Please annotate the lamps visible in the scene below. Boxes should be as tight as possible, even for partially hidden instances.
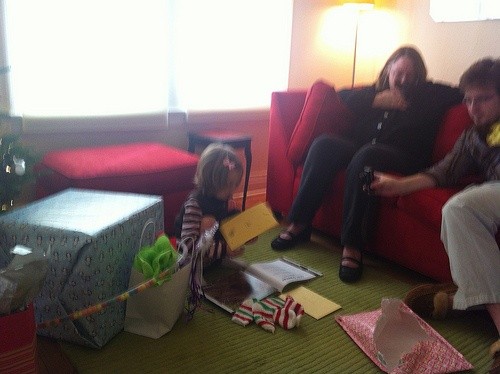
[342,0,376,89]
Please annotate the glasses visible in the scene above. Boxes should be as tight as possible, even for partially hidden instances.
[459,93,496,106]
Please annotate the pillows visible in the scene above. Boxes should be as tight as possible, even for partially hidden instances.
[287,79,356,164]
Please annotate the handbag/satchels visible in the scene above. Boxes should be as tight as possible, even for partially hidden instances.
[0,305,37,374]
[123,219,194,338]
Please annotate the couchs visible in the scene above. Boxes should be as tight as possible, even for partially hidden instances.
[266,79,476,286]
[33,142,199,242]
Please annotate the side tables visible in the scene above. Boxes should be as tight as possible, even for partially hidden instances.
[187,128,253,212]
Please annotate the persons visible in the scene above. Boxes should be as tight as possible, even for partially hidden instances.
[269,47,464,284]
[170,143,259,271]
[371,57,500,374]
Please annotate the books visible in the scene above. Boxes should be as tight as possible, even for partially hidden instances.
[198,254,322,313]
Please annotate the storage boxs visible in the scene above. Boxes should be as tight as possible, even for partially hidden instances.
[0,186,164,351]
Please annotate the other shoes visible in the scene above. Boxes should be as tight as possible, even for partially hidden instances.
[338,253,364,282]
[271,226,310,250]
